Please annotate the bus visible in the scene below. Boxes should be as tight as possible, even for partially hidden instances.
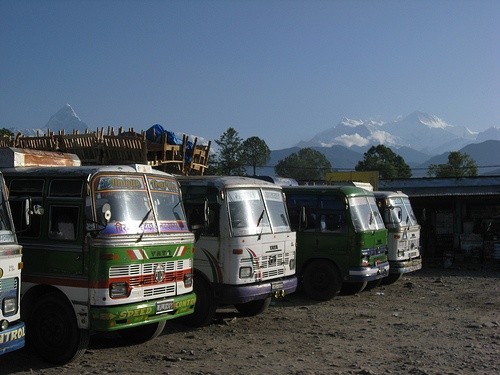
[0,173,26,355]
[179,175,297,317]
[0,146,196,365]
[245,176,390,296]
[373,191,422,283]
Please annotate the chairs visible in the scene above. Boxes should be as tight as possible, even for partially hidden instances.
[0,126,212,177]
